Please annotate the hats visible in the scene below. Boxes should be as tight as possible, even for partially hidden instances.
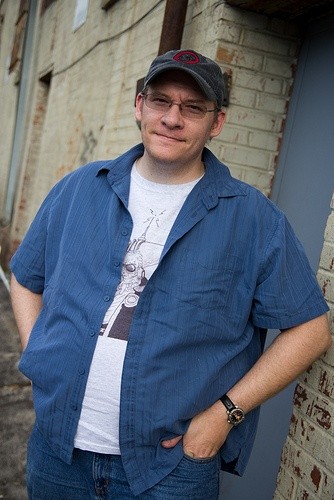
[143,48,226,112]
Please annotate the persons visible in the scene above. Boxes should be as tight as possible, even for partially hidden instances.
[9,50,332,499]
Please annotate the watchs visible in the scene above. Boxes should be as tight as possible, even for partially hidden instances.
[220,395,245,425]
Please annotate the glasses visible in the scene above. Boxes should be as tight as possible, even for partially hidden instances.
[142,91,222,120]
